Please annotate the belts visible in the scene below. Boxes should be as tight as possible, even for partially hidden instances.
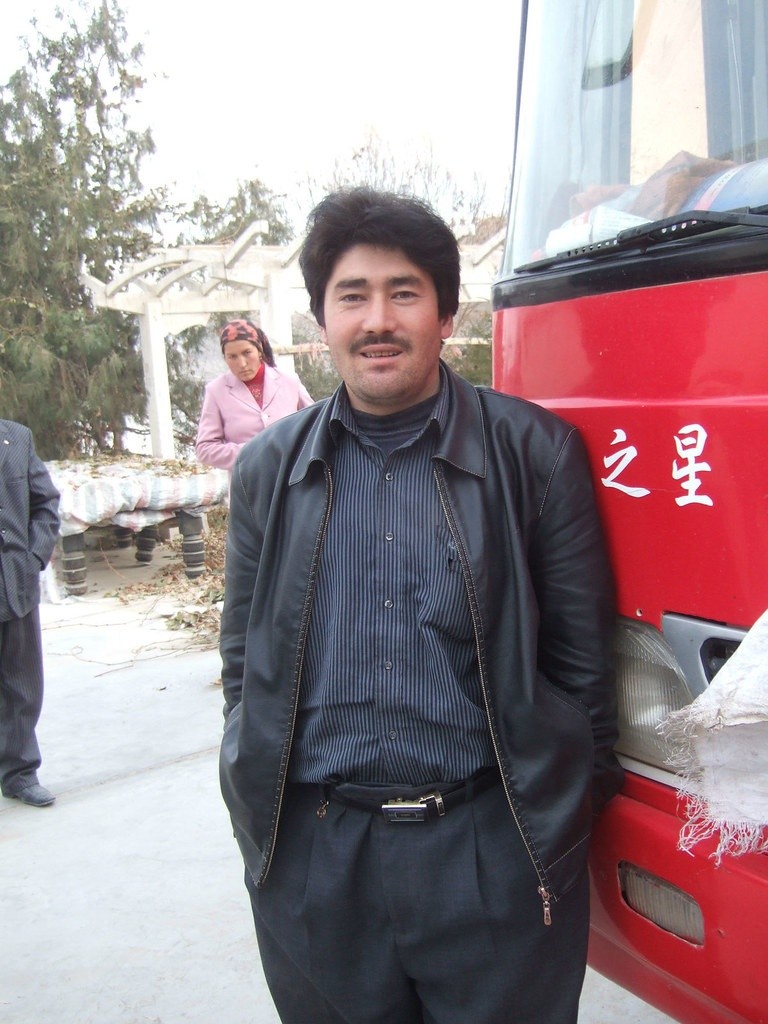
[324,764,502,822]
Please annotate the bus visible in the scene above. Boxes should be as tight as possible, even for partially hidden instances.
[491,2,768,1024]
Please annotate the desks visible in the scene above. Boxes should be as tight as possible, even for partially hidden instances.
[45,449,228,597]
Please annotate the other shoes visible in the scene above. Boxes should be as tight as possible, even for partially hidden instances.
[3,783,56,807]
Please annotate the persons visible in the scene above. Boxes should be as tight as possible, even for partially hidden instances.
[0,418,60,806]
[195,320,314,508]
[195,185,625,1024]
